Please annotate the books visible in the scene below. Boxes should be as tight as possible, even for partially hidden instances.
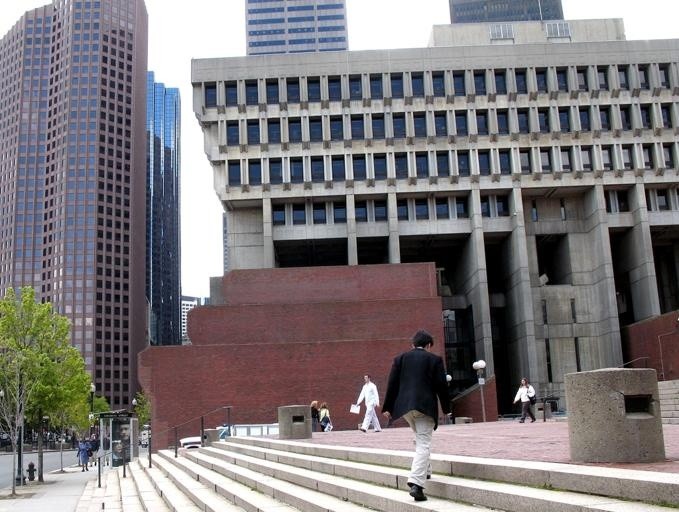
[349,403,361,415]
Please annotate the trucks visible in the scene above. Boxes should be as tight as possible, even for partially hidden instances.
[179,423,279,450]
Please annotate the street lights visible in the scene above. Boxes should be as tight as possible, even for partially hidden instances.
[88,382,96,413]
[0,390,5,402]
[88,413,94,437]
[132,397,138,412]
[472,360,488,422]
[445,373,456,424]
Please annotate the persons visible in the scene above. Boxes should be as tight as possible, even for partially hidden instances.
[513,377,537,423]
[318,402,333,432]
[355,373,383,433]
[120,422,130,441]
[70,431,111,472]
[112,439,124,461]
[381,329,454,502]
[310,400,320,432]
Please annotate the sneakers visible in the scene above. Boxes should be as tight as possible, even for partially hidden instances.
[361,428,380,432]
[410,486,426,501]
[531,418,536,422]
[427,475,430,479]
[82,462,96,471]
[520,419,524,423]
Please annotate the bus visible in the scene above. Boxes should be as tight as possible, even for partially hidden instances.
[139,424,151,447]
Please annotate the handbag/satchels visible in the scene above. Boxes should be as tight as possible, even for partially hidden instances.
[323,416,329,423]
[87,449,93,457]
[530,395,536,404]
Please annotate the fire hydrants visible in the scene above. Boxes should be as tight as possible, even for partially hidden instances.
[25,462,37,481]
